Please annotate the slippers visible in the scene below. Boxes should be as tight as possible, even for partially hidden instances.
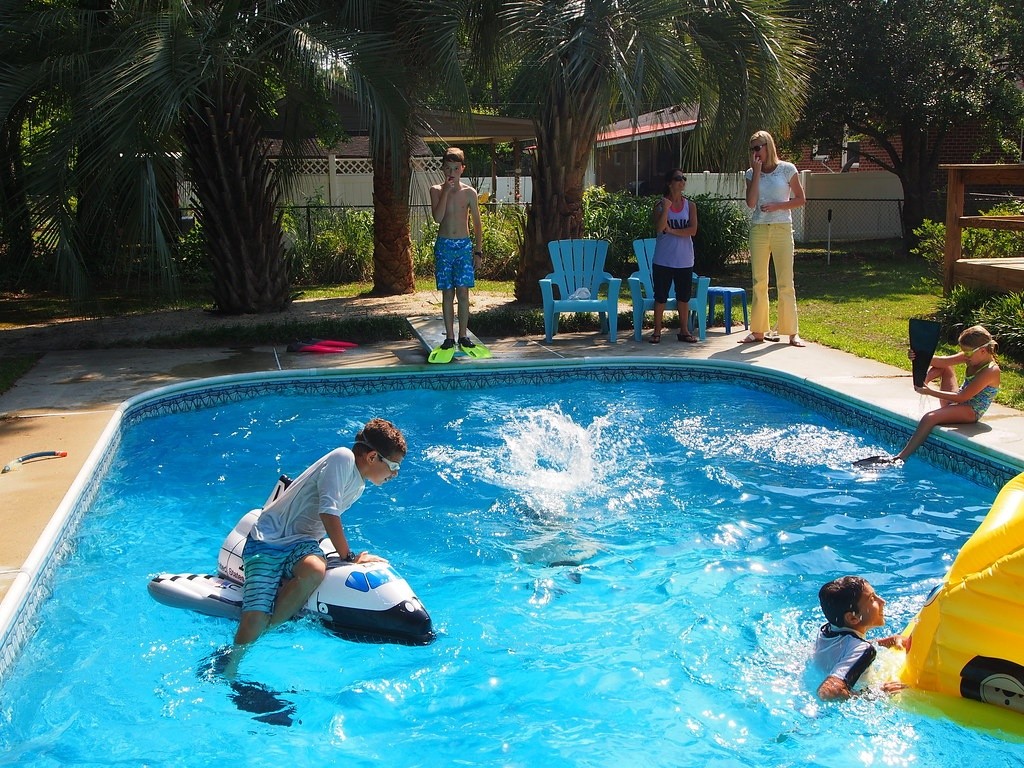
[678,335,697,343]
[649,334,660,343]
[763,330,780,341]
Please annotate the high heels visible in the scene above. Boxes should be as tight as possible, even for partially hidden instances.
[789,334,806,346]
[737,330,763,343]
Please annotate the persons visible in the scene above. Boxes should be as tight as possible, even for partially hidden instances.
[813,576,905,700]
[428,147,483,350]
[649,169,697,343]
[742,131,806,347]
[894,326,1000,462]
[222,418,407,674]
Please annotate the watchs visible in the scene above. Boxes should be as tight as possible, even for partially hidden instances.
[343,552,355,562]
[474,251,483,258]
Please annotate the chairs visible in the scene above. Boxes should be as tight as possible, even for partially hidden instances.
[538,238,623,344]
[627,238,711,344]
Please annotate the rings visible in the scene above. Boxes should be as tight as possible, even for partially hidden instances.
[766,209,768,212]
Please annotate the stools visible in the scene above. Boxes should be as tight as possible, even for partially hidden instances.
[691,286,749,335]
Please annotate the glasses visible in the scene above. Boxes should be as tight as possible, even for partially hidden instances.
[962,342,986,357]
[382,457,400,471]
[751,143,766,153]
[671,175,686,182]
